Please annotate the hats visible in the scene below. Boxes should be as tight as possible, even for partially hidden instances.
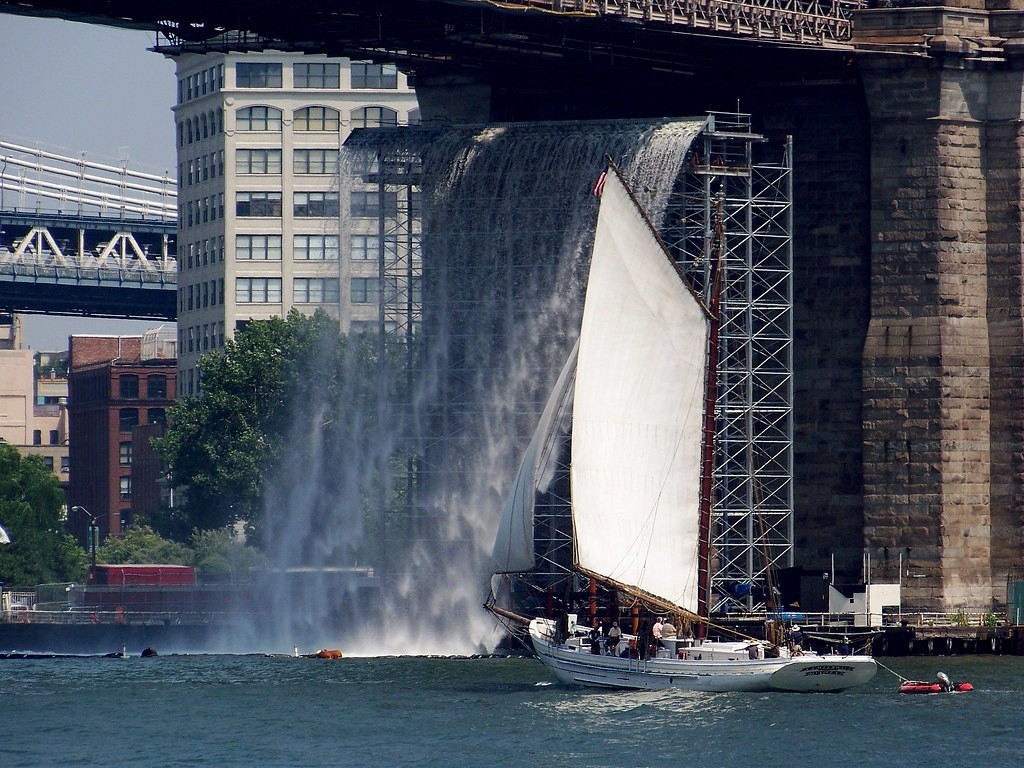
[792,624,800,631]
[571,621,574,624]
[613,622,617,626]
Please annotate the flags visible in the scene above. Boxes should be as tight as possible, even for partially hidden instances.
[592,166,609,197]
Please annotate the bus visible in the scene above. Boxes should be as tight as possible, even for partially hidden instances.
[84,563,195,586]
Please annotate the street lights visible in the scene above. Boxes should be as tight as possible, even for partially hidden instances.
[71,504,120,587]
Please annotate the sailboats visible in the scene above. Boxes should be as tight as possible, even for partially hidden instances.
[481,165,884,696]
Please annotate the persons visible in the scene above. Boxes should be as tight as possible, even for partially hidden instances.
[603,622,621,657]
[890,615,895,622]
[735,640,758,659]
[589,625,600,655]
[662,618,677,637]
[791,625,803,647]
[570,621,576,634]
[653,617,663,647]
[900,618,910,626]
